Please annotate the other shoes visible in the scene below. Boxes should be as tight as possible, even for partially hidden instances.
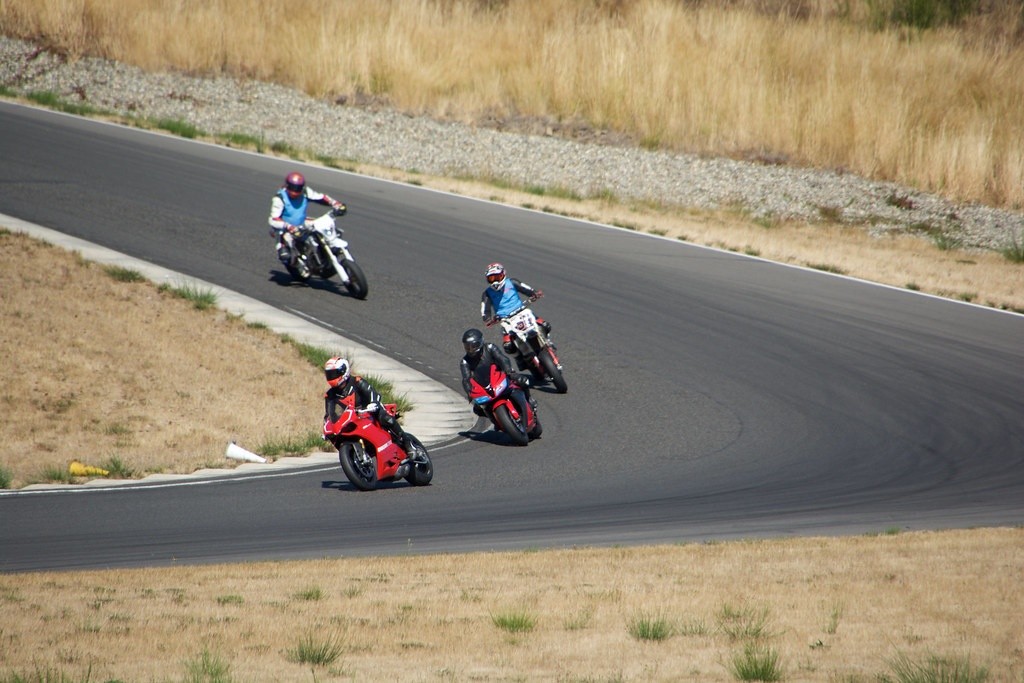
[295,258,310,277]
[526,395,538,407]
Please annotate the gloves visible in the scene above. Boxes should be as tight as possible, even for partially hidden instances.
[287,224,297,233]
[333,202,346,214]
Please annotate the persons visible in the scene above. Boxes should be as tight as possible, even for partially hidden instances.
[268,172,347,278]
[480,263,557,369]
[459,329,537,432]
[323,355,418,462]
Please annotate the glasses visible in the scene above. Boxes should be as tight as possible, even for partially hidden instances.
[489,272,504,283]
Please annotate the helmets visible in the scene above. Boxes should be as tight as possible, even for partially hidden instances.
[462,329,483,358]
[484,262,507,291]
[324,356,350,387]
[286,172,304,197]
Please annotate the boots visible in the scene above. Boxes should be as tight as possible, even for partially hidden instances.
[389,422,417,460]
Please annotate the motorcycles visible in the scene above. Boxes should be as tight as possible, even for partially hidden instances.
[269,206,368,300]
[487,291,568,395]
[322,406,433,492]
[467,360,543,446]
